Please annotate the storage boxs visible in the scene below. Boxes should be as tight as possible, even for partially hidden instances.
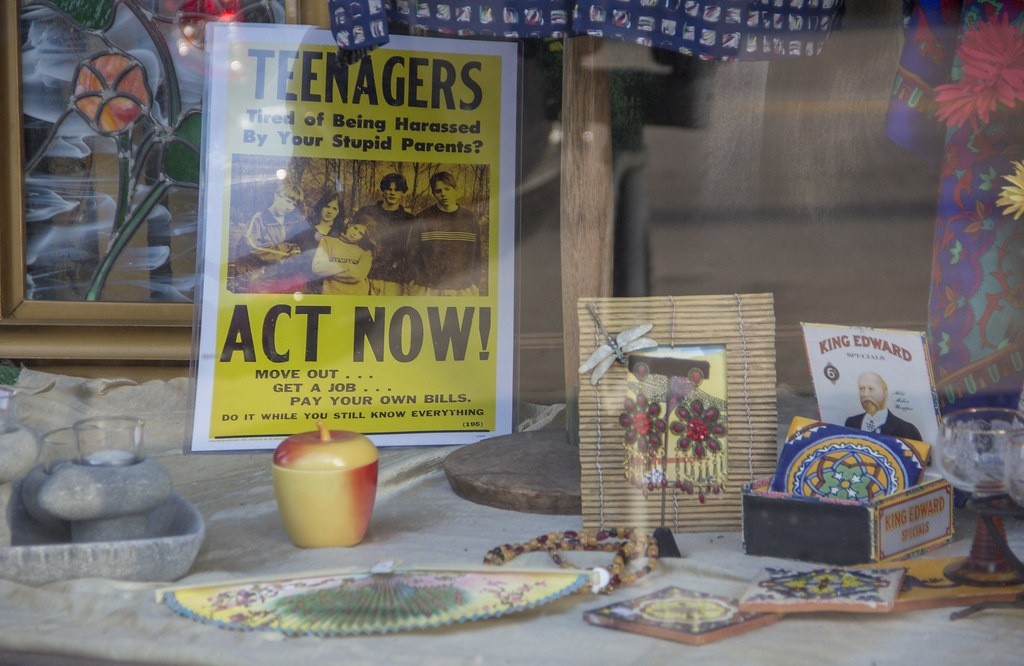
[742,473,956,568]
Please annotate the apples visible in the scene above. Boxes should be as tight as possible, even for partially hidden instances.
[271,419,379,549]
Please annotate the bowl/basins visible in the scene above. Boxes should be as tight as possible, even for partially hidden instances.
[0,478,206,587]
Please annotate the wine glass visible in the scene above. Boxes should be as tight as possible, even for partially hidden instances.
[934,407,1024,587]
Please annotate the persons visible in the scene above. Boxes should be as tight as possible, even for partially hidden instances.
[235,172,480,298]
[845,372,932,466]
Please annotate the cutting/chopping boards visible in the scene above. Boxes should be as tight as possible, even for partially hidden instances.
[442,429,582,515]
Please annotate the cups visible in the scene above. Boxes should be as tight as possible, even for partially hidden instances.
[271,431,380,548]
[40,415,146,475]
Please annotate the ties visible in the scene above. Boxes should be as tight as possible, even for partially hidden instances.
[868,418,876,430]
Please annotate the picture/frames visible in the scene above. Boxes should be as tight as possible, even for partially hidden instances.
[0,0,335,368]
